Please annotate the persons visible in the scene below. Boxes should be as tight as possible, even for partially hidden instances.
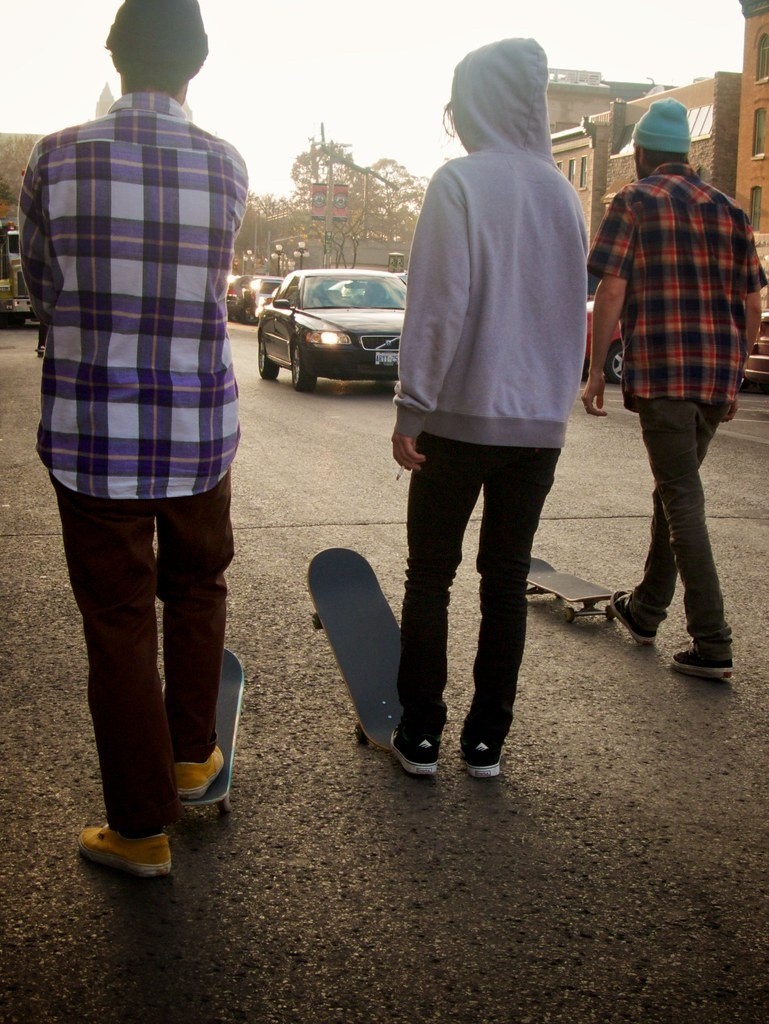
[390,38,583,779]
[583,103,762,679]
[20,0,249,878]
[34,321,49,359]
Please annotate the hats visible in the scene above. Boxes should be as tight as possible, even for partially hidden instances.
[632,98,690,153]
[106,0,205,67]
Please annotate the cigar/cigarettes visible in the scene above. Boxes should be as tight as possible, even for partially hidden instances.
[395,465,406,482]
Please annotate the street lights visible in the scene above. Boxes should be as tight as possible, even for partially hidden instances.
[271,243,291,277]
[293,240,311,270]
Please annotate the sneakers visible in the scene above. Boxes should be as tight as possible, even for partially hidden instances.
[78,828,170,876]
[460,745,501,777]
[173,745,223,800]
[611,590,656,643]
[671,640,733,678]
[391,727,437,774]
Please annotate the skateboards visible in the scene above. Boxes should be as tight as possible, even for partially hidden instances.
[525,558,620,623]
[163,650,243,814]
[305,549,402,752]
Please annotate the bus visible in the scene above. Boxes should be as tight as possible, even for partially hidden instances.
[0,220,39,328]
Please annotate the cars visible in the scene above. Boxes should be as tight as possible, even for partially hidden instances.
[225,271,410,325]
[745,310,769,395]
[580,300,624,384]
[256,268,415,394]
